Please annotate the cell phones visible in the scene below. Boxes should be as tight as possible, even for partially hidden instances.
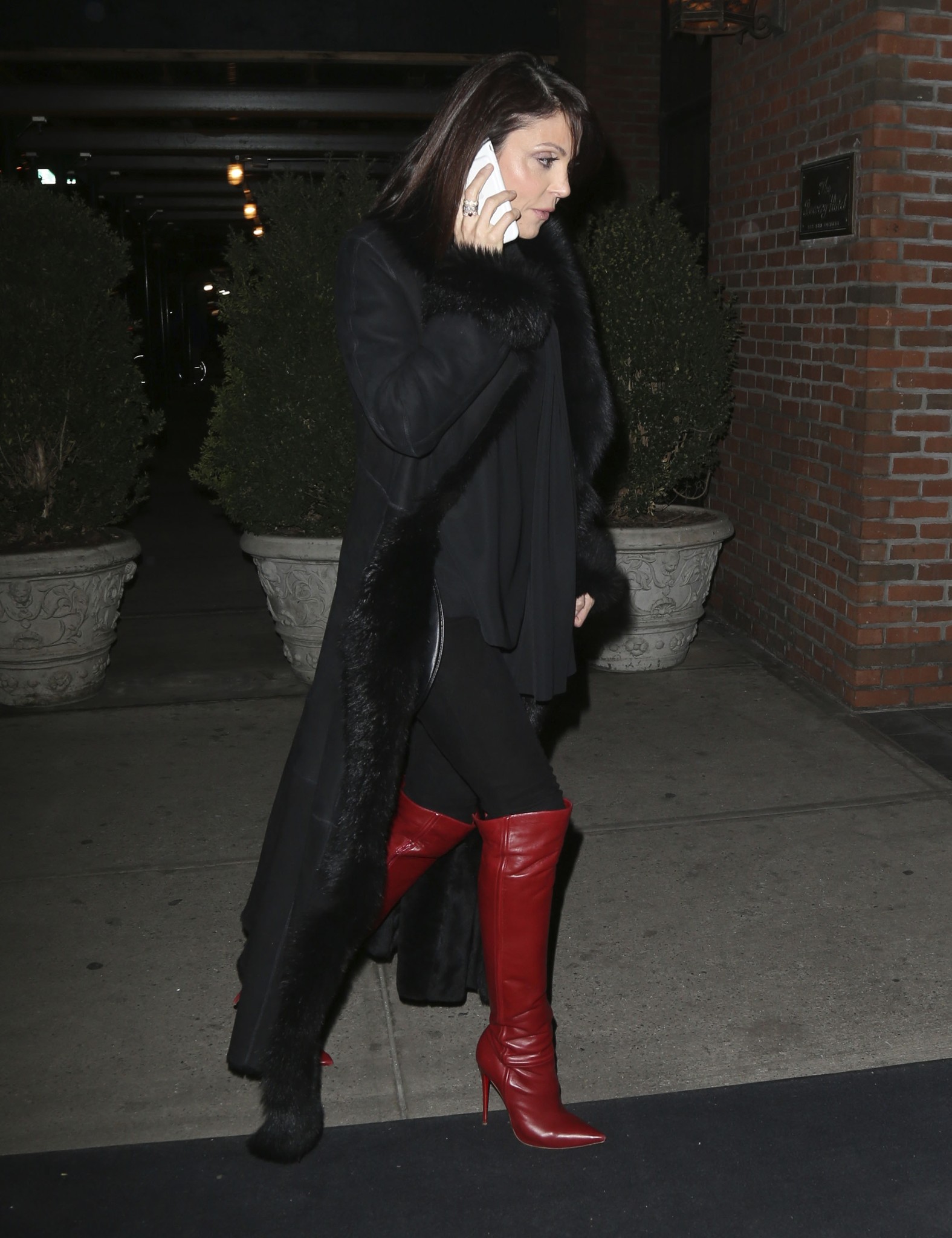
[466,136,519,244]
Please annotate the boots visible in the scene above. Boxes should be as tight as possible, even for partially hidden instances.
[235,778,473,1066]
[472,798,607,1149]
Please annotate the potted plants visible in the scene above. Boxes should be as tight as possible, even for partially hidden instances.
[0,168,167,708]
[574,178,742,671]
[190,150,378,686]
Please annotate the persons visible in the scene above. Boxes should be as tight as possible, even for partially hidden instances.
[223,51,636,1163]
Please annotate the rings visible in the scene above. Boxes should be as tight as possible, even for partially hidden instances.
[463,200,479,216]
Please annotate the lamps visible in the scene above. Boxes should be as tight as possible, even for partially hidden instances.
[665,0,787,45]
[253,226,264,239]
[227,162,244,185]
[243,201,257,220]
